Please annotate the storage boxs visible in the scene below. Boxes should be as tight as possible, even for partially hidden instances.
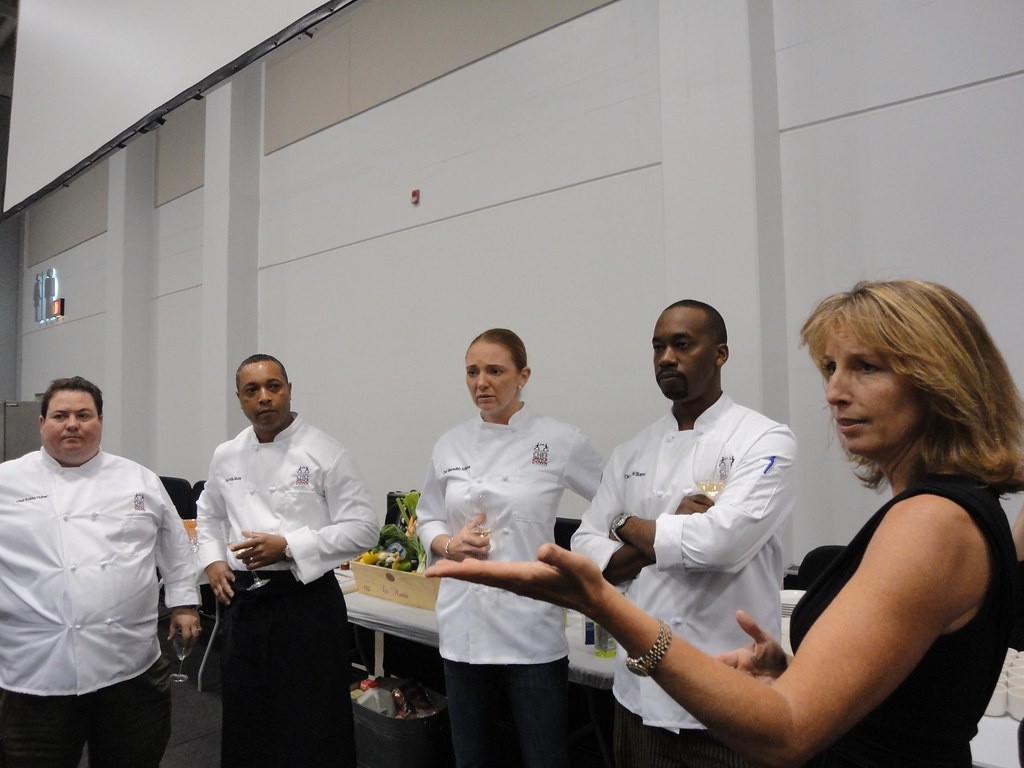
[350,555,442,612]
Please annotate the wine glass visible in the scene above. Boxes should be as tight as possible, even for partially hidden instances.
[465,494,493,559]
[221,515,271,591]
[168,627,197,684]
[693,438,732,503]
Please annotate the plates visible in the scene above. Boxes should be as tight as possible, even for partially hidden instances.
[779,590,807,618]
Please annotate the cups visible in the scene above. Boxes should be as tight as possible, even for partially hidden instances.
[985,647,1024,722]
[186,527,199,555]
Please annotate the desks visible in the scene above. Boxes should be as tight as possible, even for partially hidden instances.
[332,565,1024,768]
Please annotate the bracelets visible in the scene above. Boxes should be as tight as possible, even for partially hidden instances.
[445,537,452,556]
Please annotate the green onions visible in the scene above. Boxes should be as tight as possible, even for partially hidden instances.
[396,491,427,574]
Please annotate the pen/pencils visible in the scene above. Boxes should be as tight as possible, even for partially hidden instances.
[762,456,776,474]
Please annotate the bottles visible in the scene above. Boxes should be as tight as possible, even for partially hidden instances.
[594,621,618,658]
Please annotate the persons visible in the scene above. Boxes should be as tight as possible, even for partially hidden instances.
[425,281,1024,768]
[415,329,608,768]
[563,300,797,768]
[0,374,204,768]
[189,353,382,768]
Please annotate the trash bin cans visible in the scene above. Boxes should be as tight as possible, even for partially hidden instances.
[350,677,453,768]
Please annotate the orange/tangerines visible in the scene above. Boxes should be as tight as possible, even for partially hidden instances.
[363,551,378,563]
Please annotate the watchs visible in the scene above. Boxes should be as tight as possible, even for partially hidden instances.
[626,617,673,677]
[612,511,635,542]
[284,542,293,562]
